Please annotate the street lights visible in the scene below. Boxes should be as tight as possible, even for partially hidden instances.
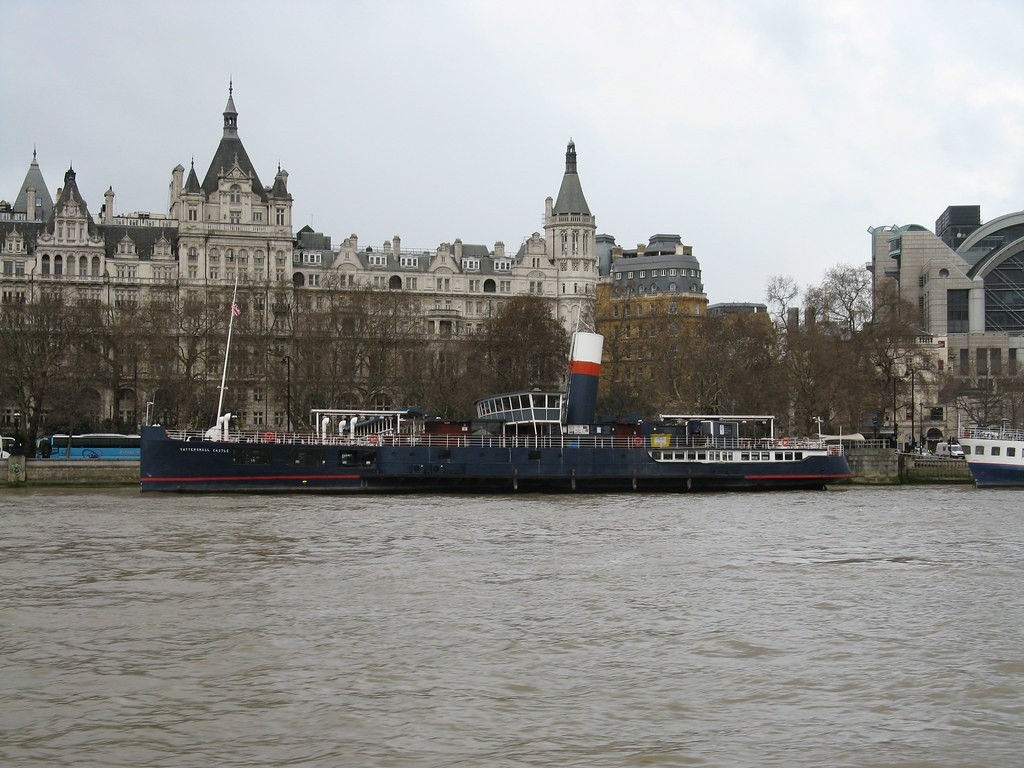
[282,356,290,432]
[893,377,903,448]
[905,368,914,452]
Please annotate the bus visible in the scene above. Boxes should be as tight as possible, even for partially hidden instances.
[0,436,23,459]
[35,433,142,460]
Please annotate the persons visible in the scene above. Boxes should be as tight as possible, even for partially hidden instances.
[152,421,161,427]
[38,435,52,458]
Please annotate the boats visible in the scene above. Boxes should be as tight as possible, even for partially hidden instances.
[957,438,1024,488]
[138,265,870,494]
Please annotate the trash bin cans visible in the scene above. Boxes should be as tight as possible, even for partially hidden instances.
[649,433,672,448]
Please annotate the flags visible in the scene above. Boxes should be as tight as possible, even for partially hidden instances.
[230,299,241,318]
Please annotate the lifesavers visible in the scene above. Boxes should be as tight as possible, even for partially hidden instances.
[370,435,379,443]
[634,437,643,446]
[782,438,789,446]
[264,431,274,442]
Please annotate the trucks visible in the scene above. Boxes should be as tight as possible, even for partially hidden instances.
[936,443,965,460]
